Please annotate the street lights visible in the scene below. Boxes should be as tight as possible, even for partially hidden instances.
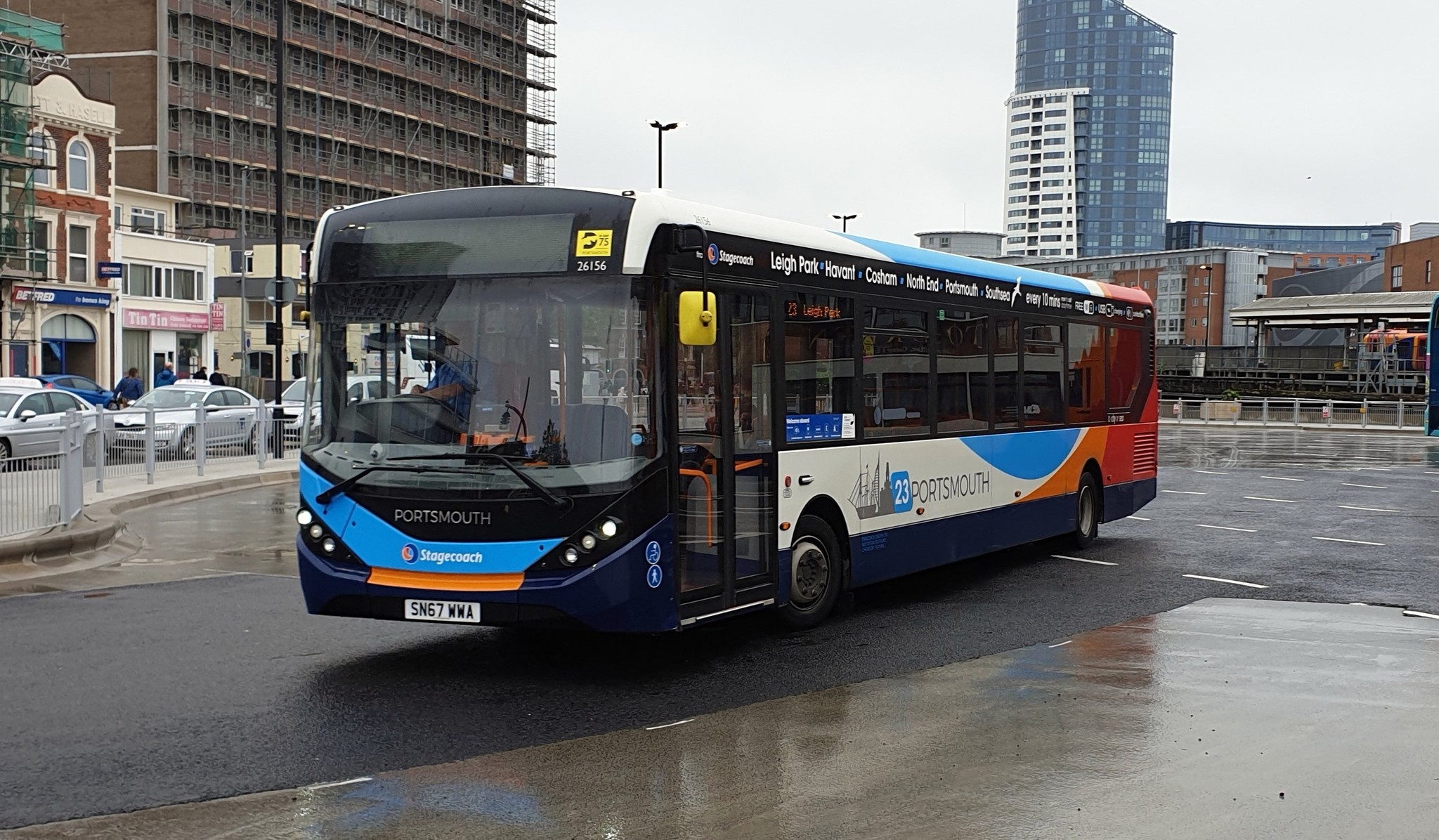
[832,214,857,233]
[1199,265,1213,394]
[648,118,680,189]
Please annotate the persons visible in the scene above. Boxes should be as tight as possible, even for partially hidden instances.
[193,366,226,386]
[111,368,144,408]
[598,377,741,412]
[410,320,498,435]
[154,359,177,388]
[733,405,764,451]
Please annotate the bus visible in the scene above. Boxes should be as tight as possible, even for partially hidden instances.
[363,331,437,395]
[1423,292,1439,438]
[1357,327,1428,393]
[293,183,1162,640]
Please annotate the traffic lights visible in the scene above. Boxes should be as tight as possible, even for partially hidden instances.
[604,360,612,374]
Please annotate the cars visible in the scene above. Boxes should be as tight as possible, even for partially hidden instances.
[0,377,119,473]
[27,374,121,411]
[262,373,411,446]
[109,376,275,463]
